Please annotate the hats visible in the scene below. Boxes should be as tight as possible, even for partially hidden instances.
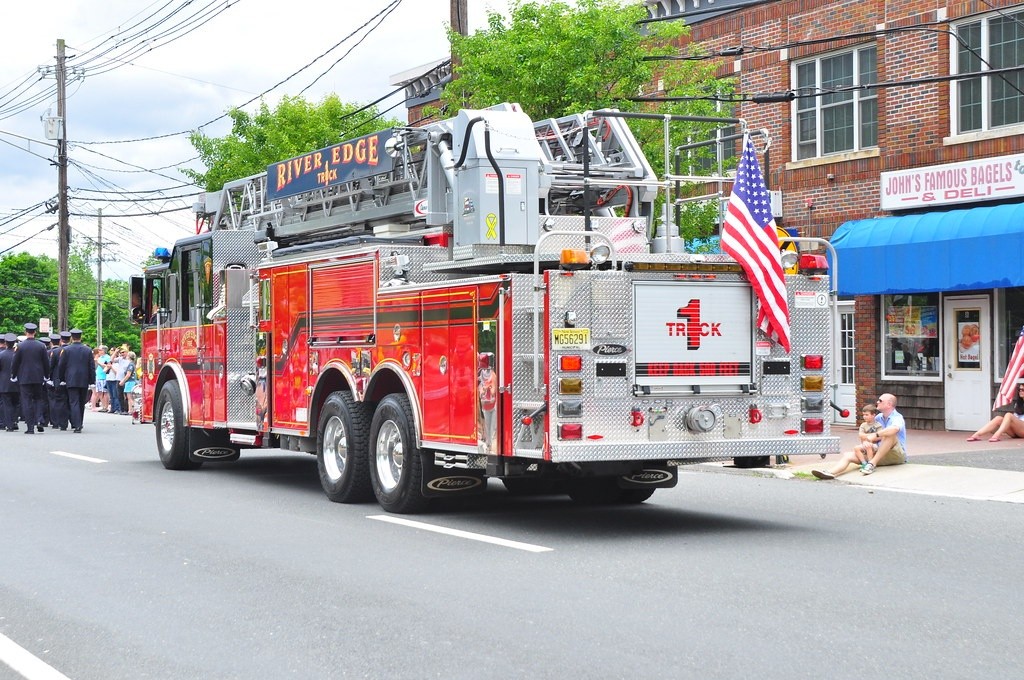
[70,329,82,336]
[39,337,51,345]
[51,334,61,341]
[17,335,28,342]
[25,323,37,331]
[0,333,5,341]
[5,333,17,344]
[60,331,71,340]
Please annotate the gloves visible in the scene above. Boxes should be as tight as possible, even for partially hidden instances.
[44,375,50,382]
[47,380,54,387]
[60,381,67,385]
[88,384,96,390]
[42,382,45,386]
[10,376,18,382]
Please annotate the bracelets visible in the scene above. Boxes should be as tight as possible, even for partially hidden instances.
[876,432,879,437]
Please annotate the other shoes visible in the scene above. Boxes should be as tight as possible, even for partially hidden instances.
[0,417,83,434]
[92,408,132,415]
[966,436,981,441]
[989,436,1002,441]
[860,461,868,472]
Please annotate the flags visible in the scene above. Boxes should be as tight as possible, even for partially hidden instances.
[720,142,791,353]
[992,328,1024,409]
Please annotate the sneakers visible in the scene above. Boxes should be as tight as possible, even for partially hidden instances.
[812,469,834,479]
[862,463,876,474]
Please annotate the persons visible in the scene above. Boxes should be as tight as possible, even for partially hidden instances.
[85,343,142,415]
[0,323,95,433]
[966,384,1024,441]
[812,394,907,479]
[853,405,882,472]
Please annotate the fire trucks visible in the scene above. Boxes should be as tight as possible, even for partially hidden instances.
[130,102,850,515]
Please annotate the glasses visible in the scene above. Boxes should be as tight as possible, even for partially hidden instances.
[879,399,887,403]
[1017,388,1024,391]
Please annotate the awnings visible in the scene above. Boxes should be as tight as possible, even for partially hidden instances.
[827,201,1024,295]
